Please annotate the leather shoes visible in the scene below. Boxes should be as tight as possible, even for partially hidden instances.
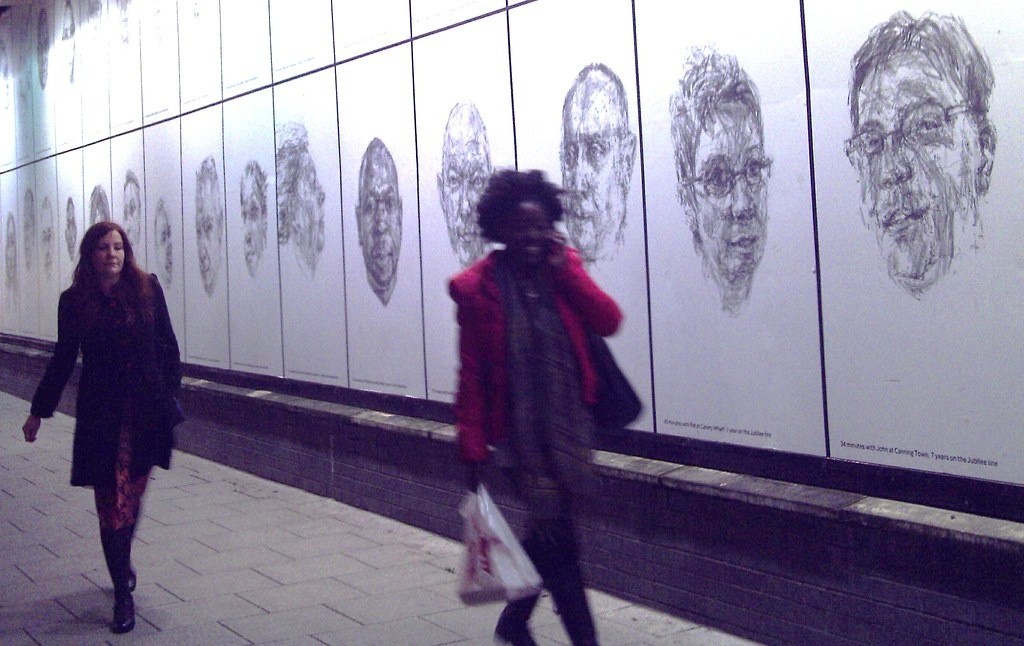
[111,594,135,635]
[127,565,136,592]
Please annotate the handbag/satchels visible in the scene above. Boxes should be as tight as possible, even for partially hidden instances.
[452,485,543,607]
[583,317,642,440]
[166,394,186,429]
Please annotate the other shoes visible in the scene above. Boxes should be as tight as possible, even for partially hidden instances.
[493,607,538,646]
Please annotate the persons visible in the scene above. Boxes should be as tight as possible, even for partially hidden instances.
[22,221,182,633]
[449,169,622,646]
